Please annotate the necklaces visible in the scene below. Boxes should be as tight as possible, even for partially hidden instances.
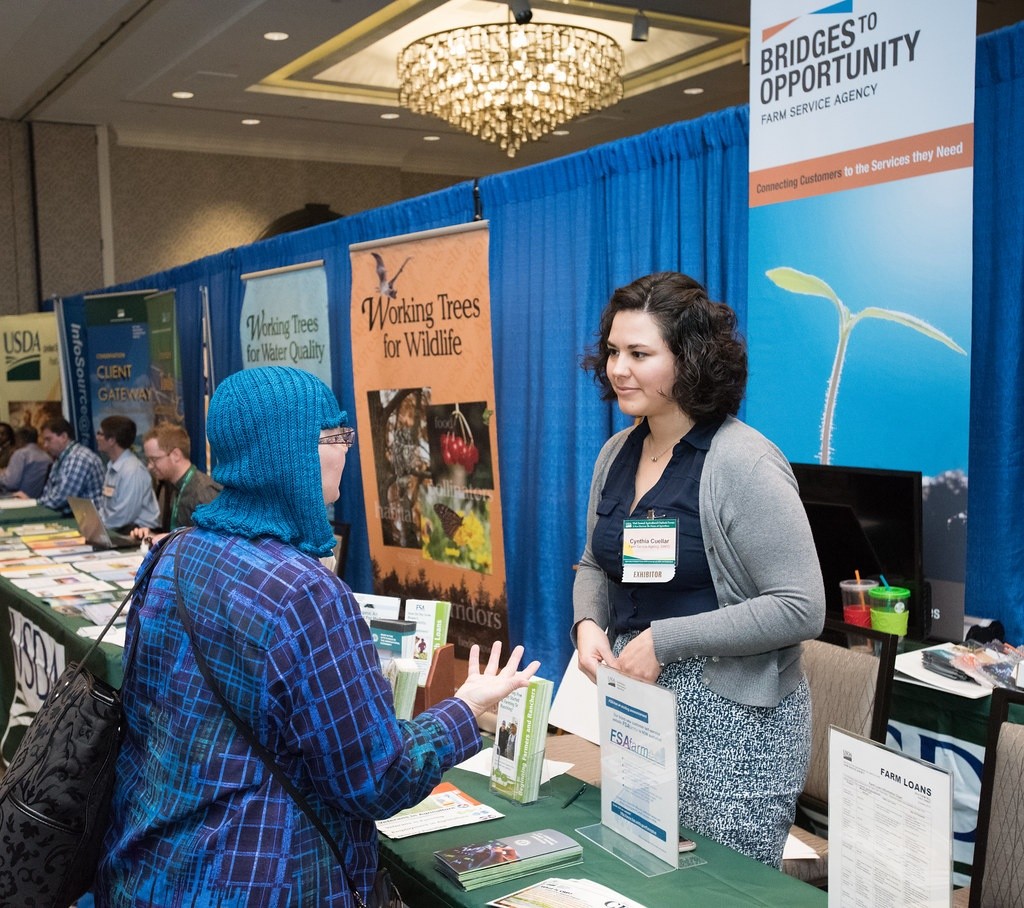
[649,425,693,462]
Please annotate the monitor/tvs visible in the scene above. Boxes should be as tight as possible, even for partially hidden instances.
[791,463,935,639]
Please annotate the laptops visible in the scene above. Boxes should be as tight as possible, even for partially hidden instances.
[66,497,143,548]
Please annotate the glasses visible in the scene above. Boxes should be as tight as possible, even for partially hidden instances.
[318,426,356,448]
[97,430,104,437]
[143,447,177,463]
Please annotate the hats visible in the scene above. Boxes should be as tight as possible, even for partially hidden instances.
[189,365,349,559]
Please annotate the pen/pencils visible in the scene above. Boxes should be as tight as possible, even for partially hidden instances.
[560,782,587,809]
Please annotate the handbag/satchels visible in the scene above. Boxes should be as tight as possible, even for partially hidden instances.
[0,662,130,908]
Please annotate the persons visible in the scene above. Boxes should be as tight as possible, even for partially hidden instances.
[0,417,106,519]
[571,271,826,870]
[92,367,541,908]
[95,415,163,536]
[130,421,224,548]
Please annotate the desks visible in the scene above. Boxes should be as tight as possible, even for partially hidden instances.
[1,493,831,907]
[856,629,1023,768]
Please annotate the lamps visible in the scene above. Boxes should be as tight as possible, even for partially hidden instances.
[398,0,628,161]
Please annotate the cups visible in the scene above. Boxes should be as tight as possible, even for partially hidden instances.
[839,580,879,656]
[869,587,911,657]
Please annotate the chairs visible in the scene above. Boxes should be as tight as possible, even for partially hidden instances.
[968,686,1023,907]
[783,620,901,887]
